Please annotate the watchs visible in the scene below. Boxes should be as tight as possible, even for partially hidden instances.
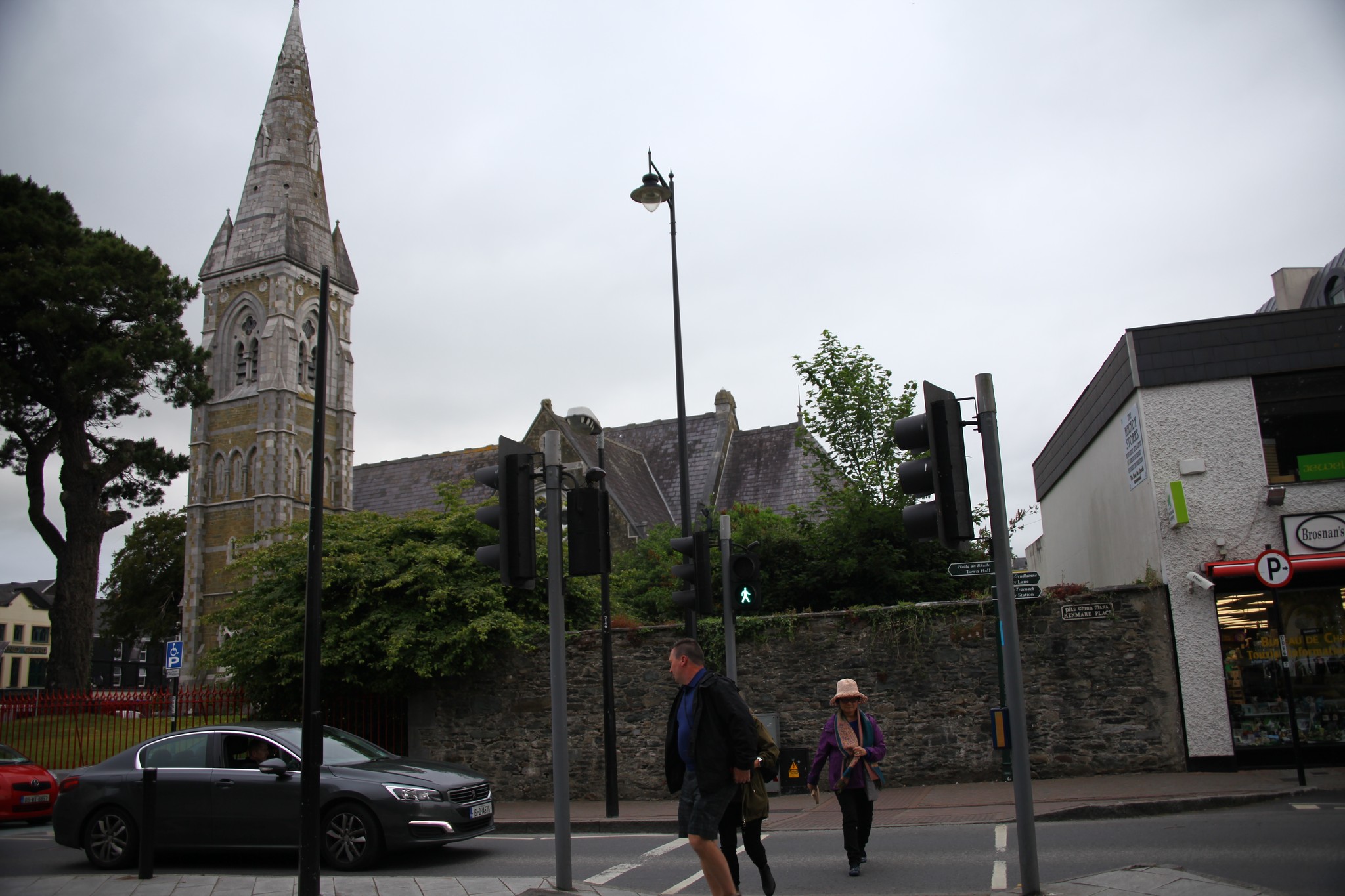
[757,756,763,767]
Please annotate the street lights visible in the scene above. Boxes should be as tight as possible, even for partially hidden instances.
[629,147,698,640]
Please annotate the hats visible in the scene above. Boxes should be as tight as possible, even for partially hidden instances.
[829,678,868,706]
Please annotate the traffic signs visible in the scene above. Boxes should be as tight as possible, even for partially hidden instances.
[947,561,996,577]
[992,584,1042,600]
[991,571,1041,588]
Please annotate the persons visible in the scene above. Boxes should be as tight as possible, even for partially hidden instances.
[719,689,779,896]
[664,638,758,896]
[241,740,269,770]
[808,679,887,875]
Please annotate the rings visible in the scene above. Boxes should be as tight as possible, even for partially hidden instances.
[858,752,859,754]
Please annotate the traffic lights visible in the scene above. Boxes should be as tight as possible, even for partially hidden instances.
[670,531,714,612]
[894,380,975,549]
[474,434,537,586]
[731,553,762,610]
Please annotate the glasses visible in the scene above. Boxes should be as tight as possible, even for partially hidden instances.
[840,698,858,703]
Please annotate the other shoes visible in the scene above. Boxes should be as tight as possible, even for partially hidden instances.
[848,866,860,876]
[861,855,867,862]
[734,882,741,895]
[758,863,775,896]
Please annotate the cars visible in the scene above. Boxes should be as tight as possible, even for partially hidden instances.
[0,743,59,829]
[51,721,497,870]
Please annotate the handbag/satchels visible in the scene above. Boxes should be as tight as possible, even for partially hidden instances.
[763,760,779,784]
[864,768,878,804]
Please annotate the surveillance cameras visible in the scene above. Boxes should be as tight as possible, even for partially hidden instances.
[1186,572,1215,591]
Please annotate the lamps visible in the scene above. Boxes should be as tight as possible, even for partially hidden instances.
[1266,487,1286,505]
